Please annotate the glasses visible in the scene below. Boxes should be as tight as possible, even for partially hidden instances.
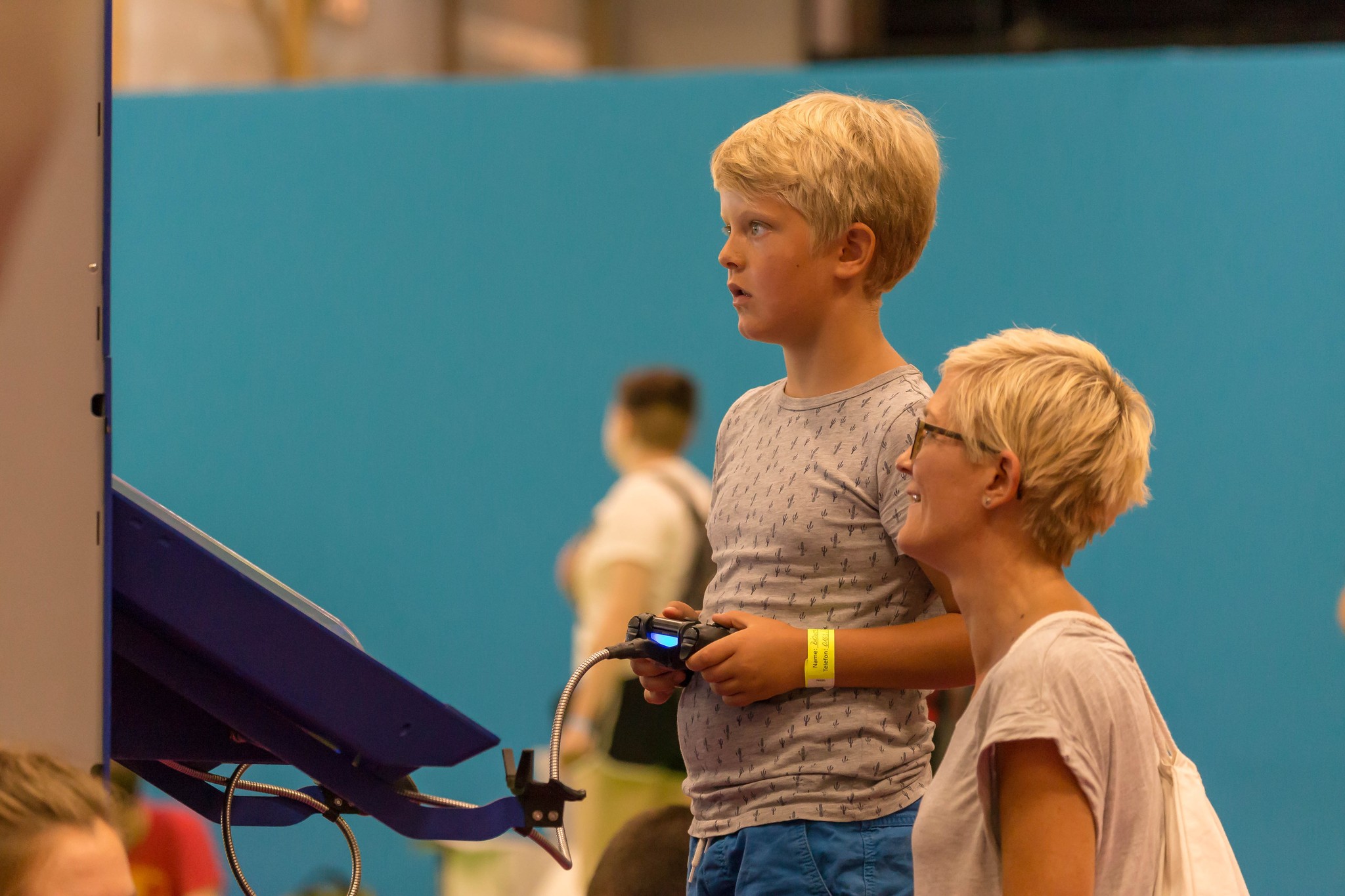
[908,418,1023,501]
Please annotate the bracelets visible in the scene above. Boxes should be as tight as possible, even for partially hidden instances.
[806,629,835,691]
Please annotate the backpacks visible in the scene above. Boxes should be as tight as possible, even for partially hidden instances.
[1004,611,1251,896]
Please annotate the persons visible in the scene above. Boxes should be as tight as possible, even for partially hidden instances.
[632,88,976,896]
[884,328,1253,896]
[559,369,715,896]
[0,736,229,896]
